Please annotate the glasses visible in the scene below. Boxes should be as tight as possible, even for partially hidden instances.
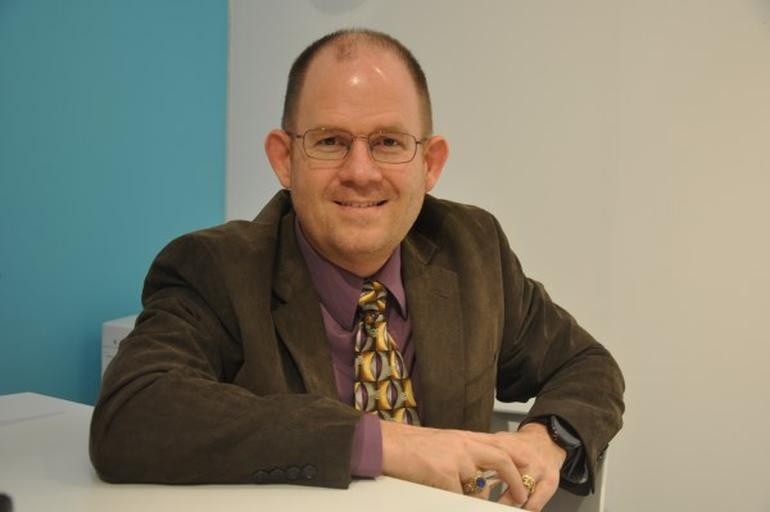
[287,128,430,163]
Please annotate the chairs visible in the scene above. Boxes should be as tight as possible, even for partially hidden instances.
[489,408,608,512]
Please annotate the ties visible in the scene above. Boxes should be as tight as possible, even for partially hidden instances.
[351,278,423,426]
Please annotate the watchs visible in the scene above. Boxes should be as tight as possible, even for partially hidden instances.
[515,416,582,449]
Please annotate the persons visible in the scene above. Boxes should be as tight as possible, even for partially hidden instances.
[88,29,625,512]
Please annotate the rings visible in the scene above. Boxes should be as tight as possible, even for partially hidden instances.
[521,475,536,495]
[460,470,489,497]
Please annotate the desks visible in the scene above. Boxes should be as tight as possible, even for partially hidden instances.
[0,390,528,512]
